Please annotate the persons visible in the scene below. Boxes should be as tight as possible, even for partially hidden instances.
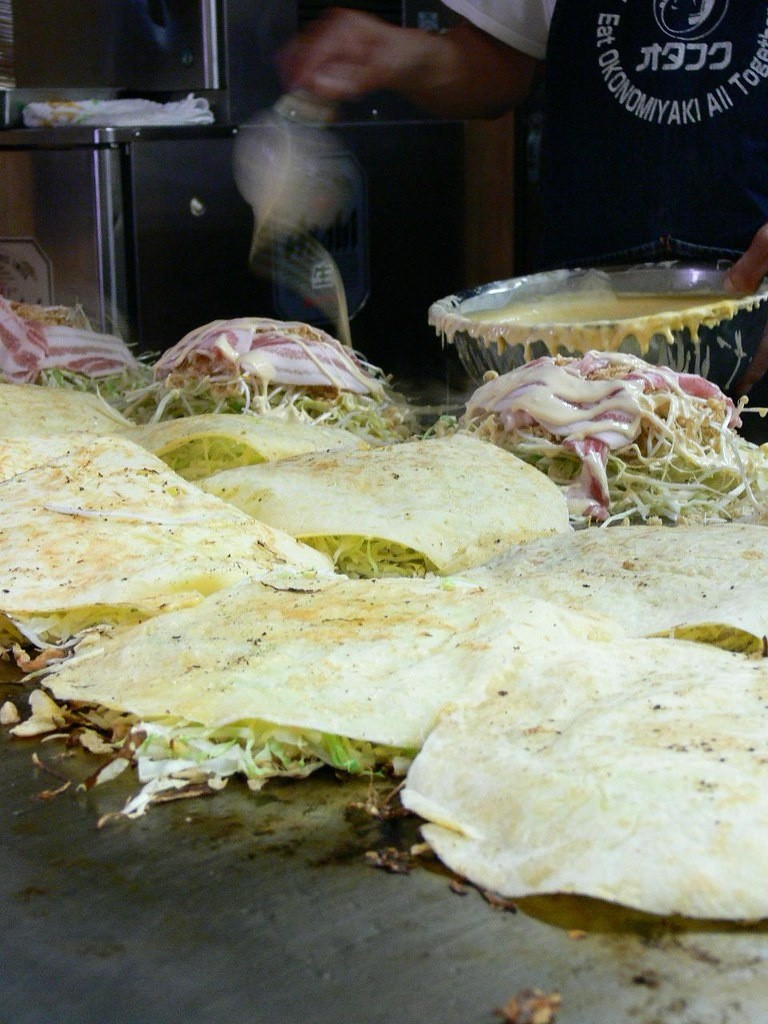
[278,0,768,444]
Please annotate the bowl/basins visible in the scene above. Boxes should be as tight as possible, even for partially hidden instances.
[430,261,767,403]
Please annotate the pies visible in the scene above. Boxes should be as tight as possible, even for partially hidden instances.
[0,381,767,926]
